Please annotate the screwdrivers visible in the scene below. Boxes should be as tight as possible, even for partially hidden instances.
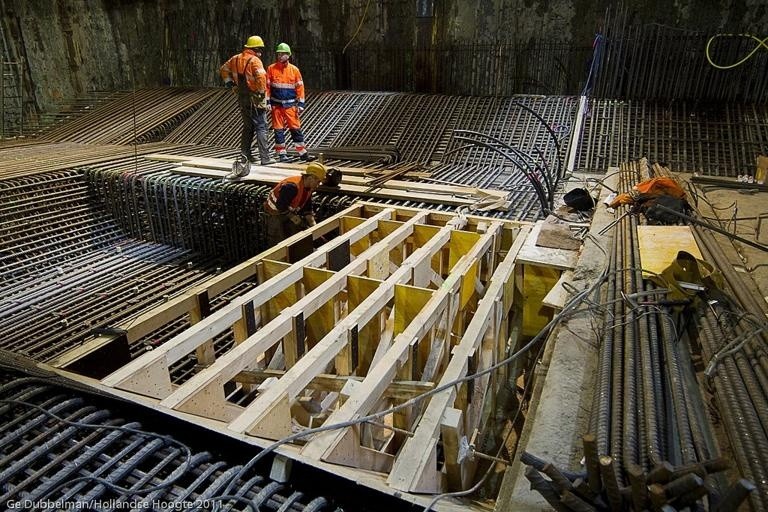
[636,301,673,306]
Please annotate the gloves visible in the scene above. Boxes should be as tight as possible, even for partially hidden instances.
[287,212,302,226]
[305,214,317,227]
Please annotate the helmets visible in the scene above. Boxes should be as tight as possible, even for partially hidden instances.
[299,162,329,184]
[275,42,292,56]
[243,35,265,49]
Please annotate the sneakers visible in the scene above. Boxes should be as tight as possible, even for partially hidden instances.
[279,152,317,163]
[242,155,277,165]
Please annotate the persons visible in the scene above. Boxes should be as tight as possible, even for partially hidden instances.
[261,161,328,251]
[264,41,314,163]
[219,33,277,166]
